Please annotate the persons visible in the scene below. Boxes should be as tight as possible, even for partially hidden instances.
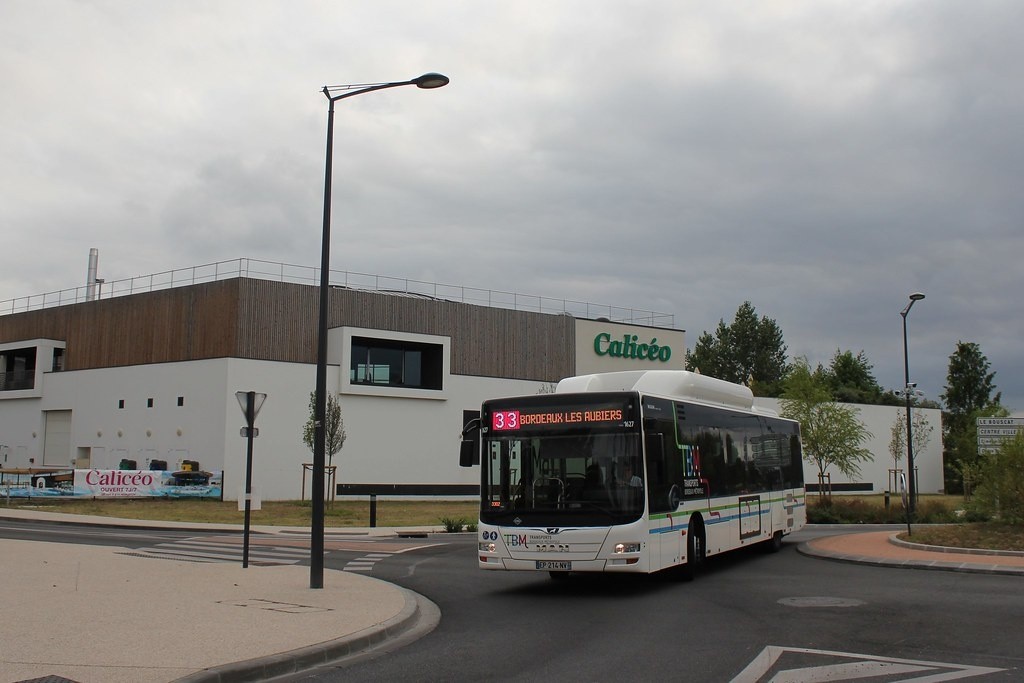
[611,462,643,488]
[743,460,763,478]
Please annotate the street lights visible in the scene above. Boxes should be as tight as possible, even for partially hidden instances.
[310,70,450,590]
[899,292,926,516]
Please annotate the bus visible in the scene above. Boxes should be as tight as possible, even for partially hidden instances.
[458,369,813,584]
